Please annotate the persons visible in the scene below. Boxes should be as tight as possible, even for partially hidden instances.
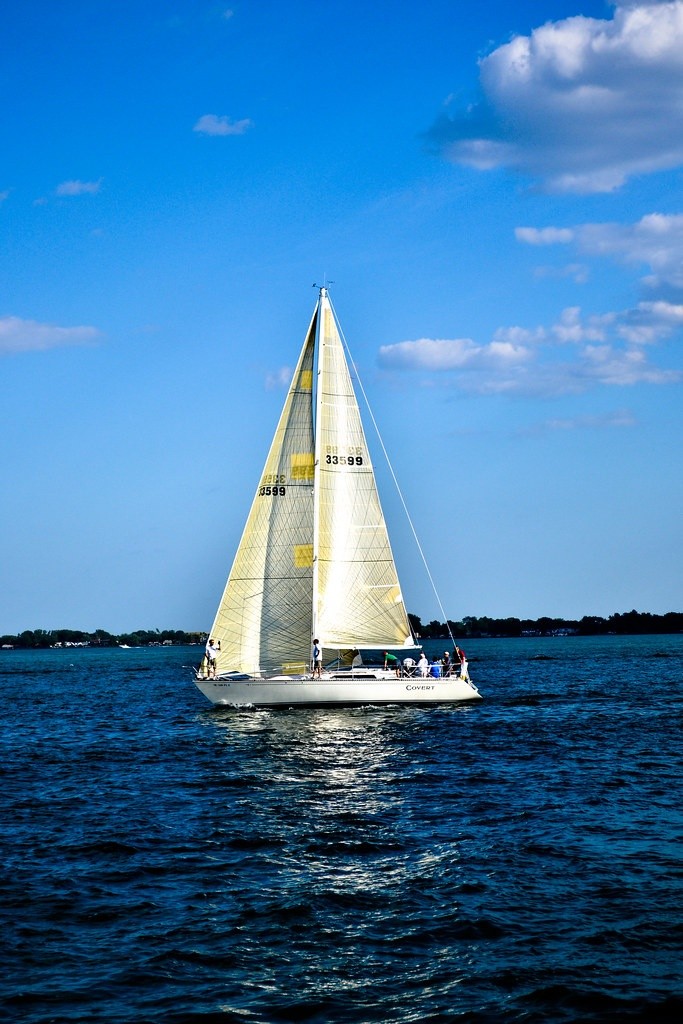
[205,639,222,680]
[310,638,323,679]
[382,651,401,678]
[403,645,465,678]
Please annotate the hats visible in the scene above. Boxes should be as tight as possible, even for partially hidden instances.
[384,652,386,654]
[444,652,449,654]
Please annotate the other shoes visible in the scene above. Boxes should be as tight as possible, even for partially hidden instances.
[206,678,210,680]
[316,677,322,679]
[311,678,314,679]
[213,677,216,680]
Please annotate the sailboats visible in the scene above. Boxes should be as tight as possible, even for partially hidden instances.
[181,272,485,706]
